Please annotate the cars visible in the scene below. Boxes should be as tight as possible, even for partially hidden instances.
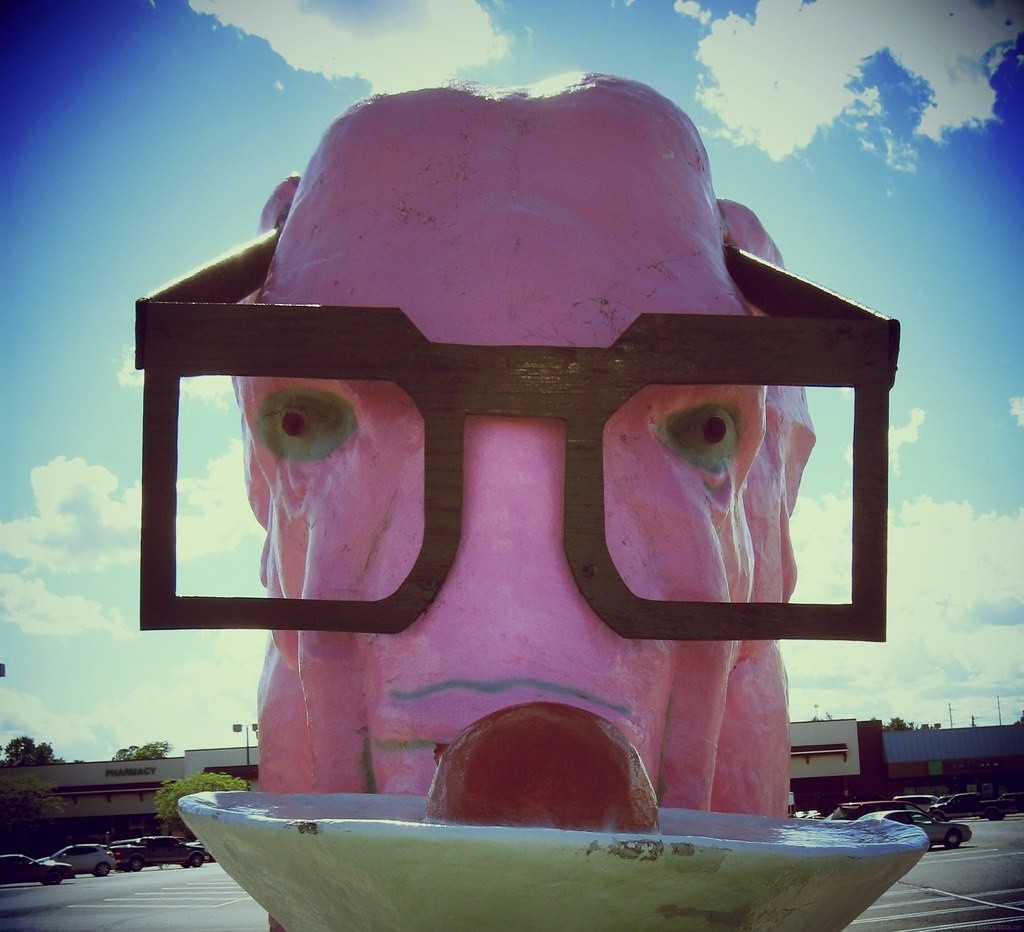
[36,844,117,877]
[831,800,945,822]
[892,794,940,814]
[0,854,75,886]
[184,838,217,862]
[999,793,1024,812]
[850,811,973,849]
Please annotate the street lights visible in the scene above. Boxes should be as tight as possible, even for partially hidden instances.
[233,724,258,791]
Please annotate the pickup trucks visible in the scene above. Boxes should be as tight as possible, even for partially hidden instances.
[111,836,205,872]
[929,792,1018,820]
